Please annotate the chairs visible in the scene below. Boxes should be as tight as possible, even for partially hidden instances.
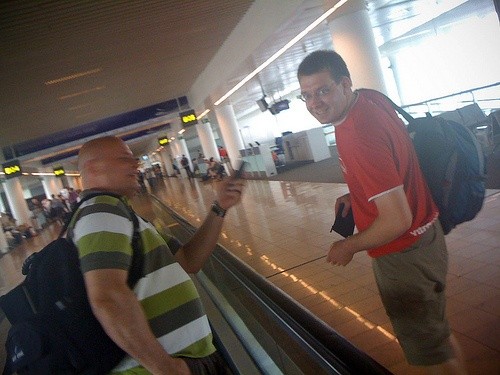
[270,137,284,154]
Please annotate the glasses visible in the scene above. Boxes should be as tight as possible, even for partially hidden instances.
[295,78,340,102]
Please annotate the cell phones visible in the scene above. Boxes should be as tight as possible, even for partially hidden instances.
[235,161,244,179]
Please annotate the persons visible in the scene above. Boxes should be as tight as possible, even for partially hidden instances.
[297,50,468,375]
[0,135,250,375]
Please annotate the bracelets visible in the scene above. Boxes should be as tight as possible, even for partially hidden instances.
[210,201,227,219]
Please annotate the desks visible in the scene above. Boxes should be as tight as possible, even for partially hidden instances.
[283,128,331,162]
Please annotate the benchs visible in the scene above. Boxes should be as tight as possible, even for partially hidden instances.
[433,103,488,145]
[488,110,500,157]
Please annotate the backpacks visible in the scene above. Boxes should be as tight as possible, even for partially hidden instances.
[0,190,146,375]
[355,88,487,234]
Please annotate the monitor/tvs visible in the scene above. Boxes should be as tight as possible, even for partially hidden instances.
[2,159,22,179]
[179,109,198,128]
[158,136,168,146]
[53,166,65,177]
